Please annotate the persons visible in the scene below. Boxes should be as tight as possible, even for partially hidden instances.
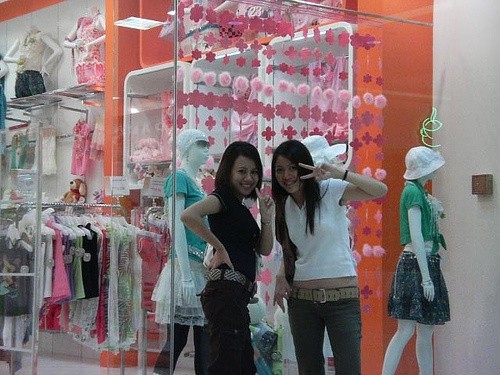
[65,7,106,85]
[271,139,388,375]
[180,141,273,375]
[382,145,451,375]
[272,135,347,375]
[181,0,236,58]
[0,55,9,130]
[151,129,210,375]
[4,24,63,97]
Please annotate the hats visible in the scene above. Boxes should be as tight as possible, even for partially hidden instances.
[403,146,445,180]
[176,129,210,157]
[301,135,347,166]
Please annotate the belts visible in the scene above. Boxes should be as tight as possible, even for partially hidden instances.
[287,286,359,303]
[208,269,257,299]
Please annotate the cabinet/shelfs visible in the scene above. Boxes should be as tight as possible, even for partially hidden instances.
[122,22,355,188]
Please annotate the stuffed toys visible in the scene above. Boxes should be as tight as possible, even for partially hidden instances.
[61,178,86,203]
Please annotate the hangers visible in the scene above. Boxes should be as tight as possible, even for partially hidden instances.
[5,200,167,252]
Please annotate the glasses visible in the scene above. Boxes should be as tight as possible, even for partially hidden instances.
[195,141,210,149]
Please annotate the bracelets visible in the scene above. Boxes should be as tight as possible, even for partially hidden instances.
[343,169,350,180]
[261,220,271,225]
